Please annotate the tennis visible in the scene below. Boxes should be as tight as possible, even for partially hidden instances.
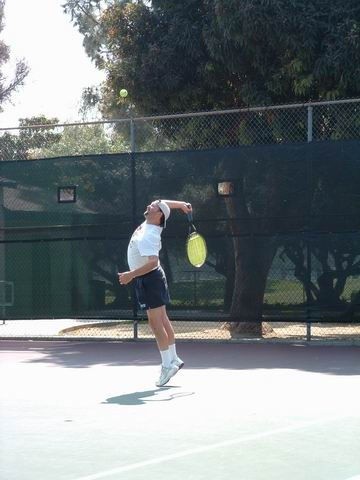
[120,89,128,97]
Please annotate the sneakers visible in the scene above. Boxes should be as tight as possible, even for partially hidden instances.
[155,365,179,386]
[172,357,184,369]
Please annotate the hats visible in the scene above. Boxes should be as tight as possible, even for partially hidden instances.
[154,200,171,229]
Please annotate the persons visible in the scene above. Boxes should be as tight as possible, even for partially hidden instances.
[117,200,194,386]
